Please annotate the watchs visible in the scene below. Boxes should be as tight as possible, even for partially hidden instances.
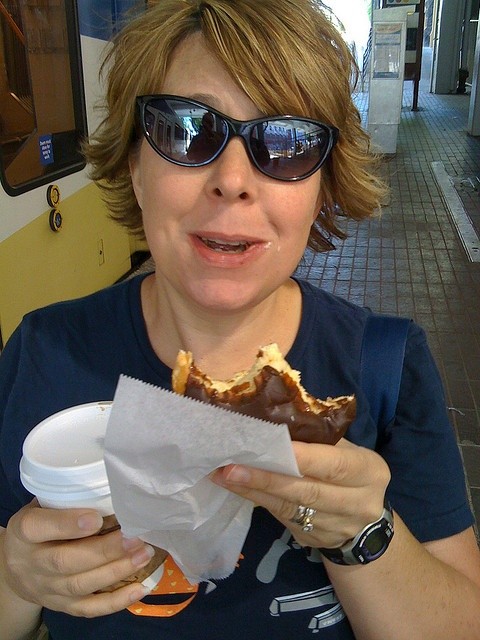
[317,497,395,566]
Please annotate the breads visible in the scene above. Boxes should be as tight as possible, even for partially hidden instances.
[171,344,359,446]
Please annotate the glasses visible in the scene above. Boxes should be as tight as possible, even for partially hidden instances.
[135,94,339,182]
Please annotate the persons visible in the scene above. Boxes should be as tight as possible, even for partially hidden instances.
[0,0,478,639]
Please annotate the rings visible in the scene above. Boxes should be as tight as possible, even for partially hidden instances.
[289,504,316,533]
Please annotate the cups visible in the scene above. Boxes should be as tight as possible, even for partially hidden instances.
[19,398,201,618]
